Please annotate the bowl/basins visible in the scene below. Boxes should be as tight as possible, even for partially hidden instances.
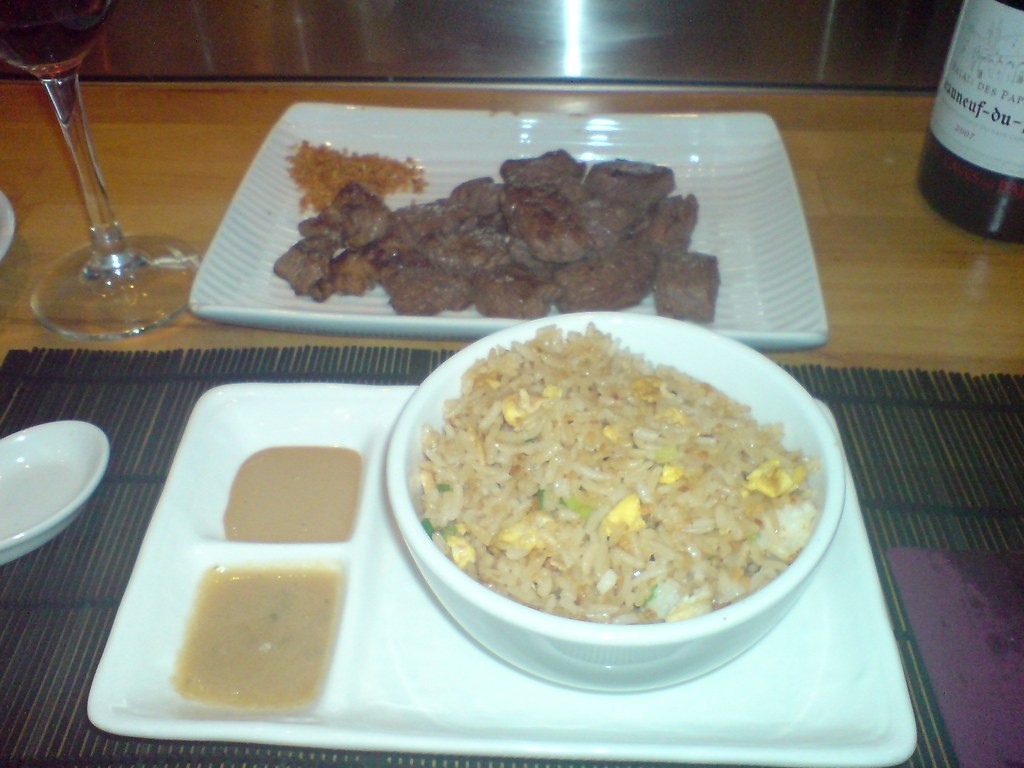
[383,310,845,696]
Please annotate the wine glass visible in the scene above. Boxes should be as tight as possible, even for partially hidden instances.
[1,1,206,335]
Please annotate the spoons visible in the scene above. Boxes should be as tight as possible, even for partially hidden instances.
[1,419,110,567]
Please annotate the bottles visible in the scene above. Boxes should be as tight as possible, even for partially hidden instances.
[920,0,1023,246]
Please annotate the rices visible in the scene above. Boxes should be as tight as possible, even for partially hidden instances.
[416,320,821,626]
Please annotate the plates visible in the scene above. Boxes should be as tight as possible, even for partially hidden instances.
[191,101,829,353]
[86,379,918,765]
